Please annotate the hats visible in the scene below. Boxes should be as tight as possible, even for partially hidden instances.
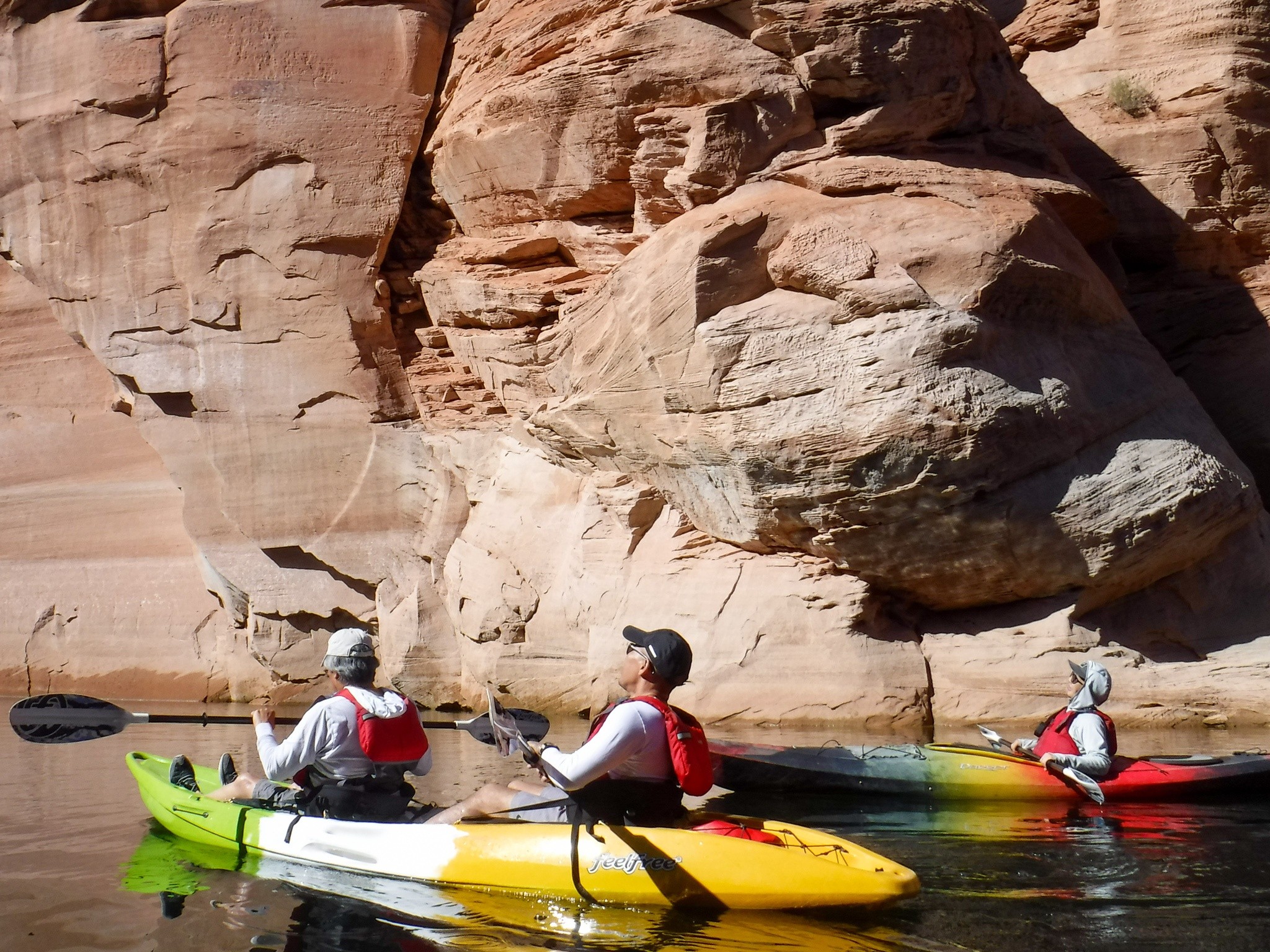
[623,624,693,685]
[1068,659,1087,680]
[320,627,377,666]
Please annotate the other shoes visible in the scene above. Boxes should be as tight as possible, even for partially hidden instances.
[323,809,340,822]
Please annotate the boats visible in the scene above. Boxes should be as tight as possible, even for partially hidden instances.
[123,744,925,918]
[705,728,1270,820]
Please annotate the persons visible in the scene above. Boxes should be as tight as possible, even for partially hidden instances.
[169,629,432,820]
[1010,660,1118,777]
[324,627,711,831]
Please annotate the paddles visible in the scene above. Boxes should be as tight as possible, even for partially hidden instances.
[976,724,1105,804]
[486,689,538,763]
[9,693,550,745]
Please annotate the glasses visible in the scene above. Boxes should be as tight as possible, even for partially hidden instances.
[1070,671,1084,686]
[627,642,655,676]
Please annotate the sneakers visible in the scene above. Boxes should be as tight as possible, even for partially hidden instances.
[169,755,200,792]
[218,751,237,787]
